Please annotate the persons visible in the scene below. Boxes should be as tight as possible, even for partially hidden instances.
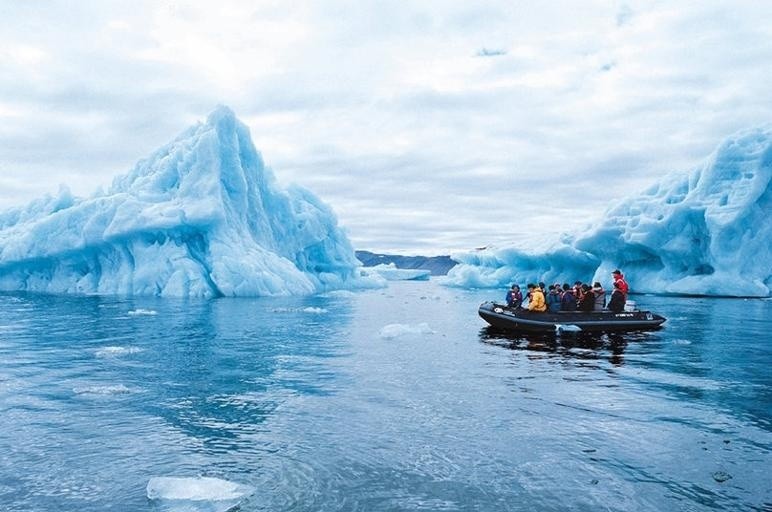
[503,269,630,313]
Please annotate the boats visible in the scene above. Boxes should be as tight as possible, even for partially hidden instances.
[480,301,668,333]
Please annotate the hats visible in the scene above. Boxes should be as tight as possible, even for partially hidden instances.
[611,270,620,274]
